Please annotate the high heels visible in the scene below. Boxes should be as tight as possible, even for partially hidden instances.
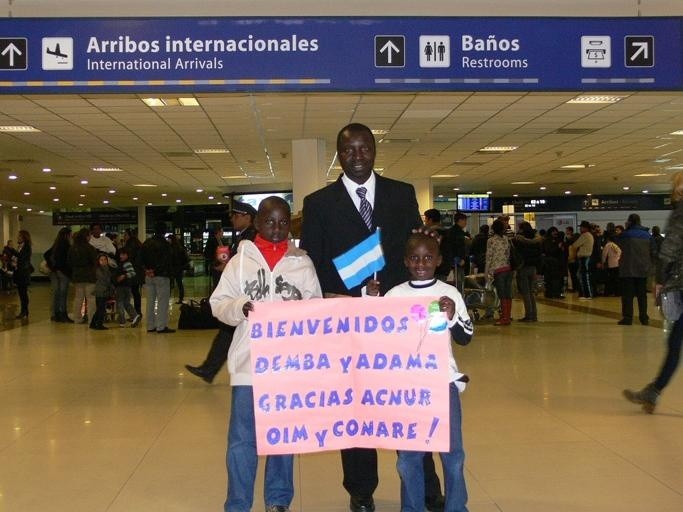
[16,310,30,319]
[128,313,144,323]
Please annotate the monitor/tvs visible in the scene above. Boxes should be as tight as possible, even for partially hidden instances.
[231,190,294,214]
[456,193,490,213]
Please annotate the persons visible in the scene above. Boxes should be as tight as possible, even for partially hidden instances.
[589,222,601,296]
[1,240,18,288]
[203,224,231,293]
[614,226,624,237]
[360,227,474,512]
[42,227,76,323]
[165,232,185,305]
[209,195,325,512]
[113,248,141,328]
[602,222,623,244]
[301,122,449,510]
[64,228,99,324]
[516,221,543,322]
[448,213,472,288]
[616,214,652,326]
[498,213,512,236]
[571,220,593,300]
[141,221,176,333]
[623,170,683,415]
[121,227,140,321]
[545,227,565,300]
[183,200,259,387]
[469,224,494,321]
[89,252,112,330]
[424,208,452,280]
[596,236,621,297]
[8,230,33,319]
[484,220,514,326]
[86,222,115,254]
[647,226,660,293]
[563,226,579,292]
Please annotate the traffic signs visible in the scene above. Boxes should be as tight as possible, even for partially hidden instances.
[0,38,28,70]
[625,35,654,68]
[374,36,404,68]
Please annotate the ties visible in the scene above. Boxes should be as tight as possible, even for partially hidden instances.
[356,186,374,233]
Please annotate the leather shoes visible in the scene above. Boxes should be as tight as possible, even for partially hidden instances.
[60,311,75,323]
[427,493,446,511]
[350,492,376,512]
[618,318,633,325]
[56,312,60,322]
[158,326,177,334]
[185,364,215,383]
[641,318,650,325]
[552,294,566,299]
[147,326,158,333]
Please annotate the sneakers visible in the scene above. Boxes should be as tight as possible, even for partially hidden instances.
[518,314,535,322]
[587,296,594,300]
[266,504,292,512]
[534,312,538,321]
[577,296,587,299]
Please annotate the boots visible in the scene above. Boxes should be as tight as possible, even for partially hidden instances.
[494,297,512,325]
[120,312,127,328]
[508,298,513,324]
[623,382,661,414]
[126,304,142,328]
[90,314,97,328]
[95,320,110,330]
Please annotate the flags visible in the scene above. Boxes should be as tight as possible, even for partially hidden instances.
[330,225,386,290]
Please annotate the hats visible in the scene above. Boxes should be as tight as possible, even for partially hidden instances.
[59,229,72,235]
[96,254,110,263]
[121,229,137,239]
[226,198,259,216]
[492,221,506,232]
[577,220,593,229]
[165,236,178,241]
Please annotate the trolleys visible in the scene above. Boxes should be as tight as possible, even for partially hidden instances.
[460,268,499,322]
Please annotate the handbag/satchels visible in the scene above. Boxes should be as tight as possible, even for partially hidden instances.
[660,285,683,320]
[44,246,66,273]
[182,248,194,269]
[178,297,219,330]
[508,239,526,272]
[210,234,232,272]
[48,272,60,299]
[30,263,36,274]
[112,263,137,290]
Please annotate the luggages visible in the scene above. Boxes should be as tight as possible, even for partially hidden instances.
[443,256,464,294]
